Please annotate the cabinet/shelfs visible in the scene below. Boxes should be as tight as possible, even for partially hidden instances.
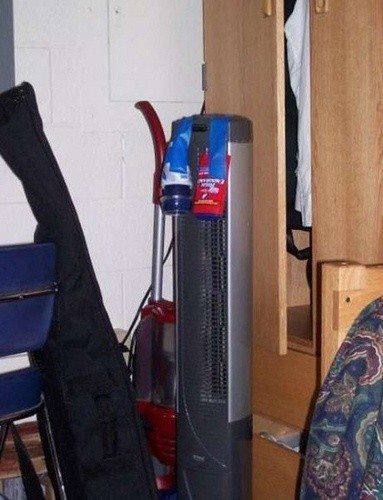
[202,0,382,500]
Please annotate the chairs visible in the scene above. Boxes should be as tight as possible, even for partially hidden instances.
[0,243,68,499]
